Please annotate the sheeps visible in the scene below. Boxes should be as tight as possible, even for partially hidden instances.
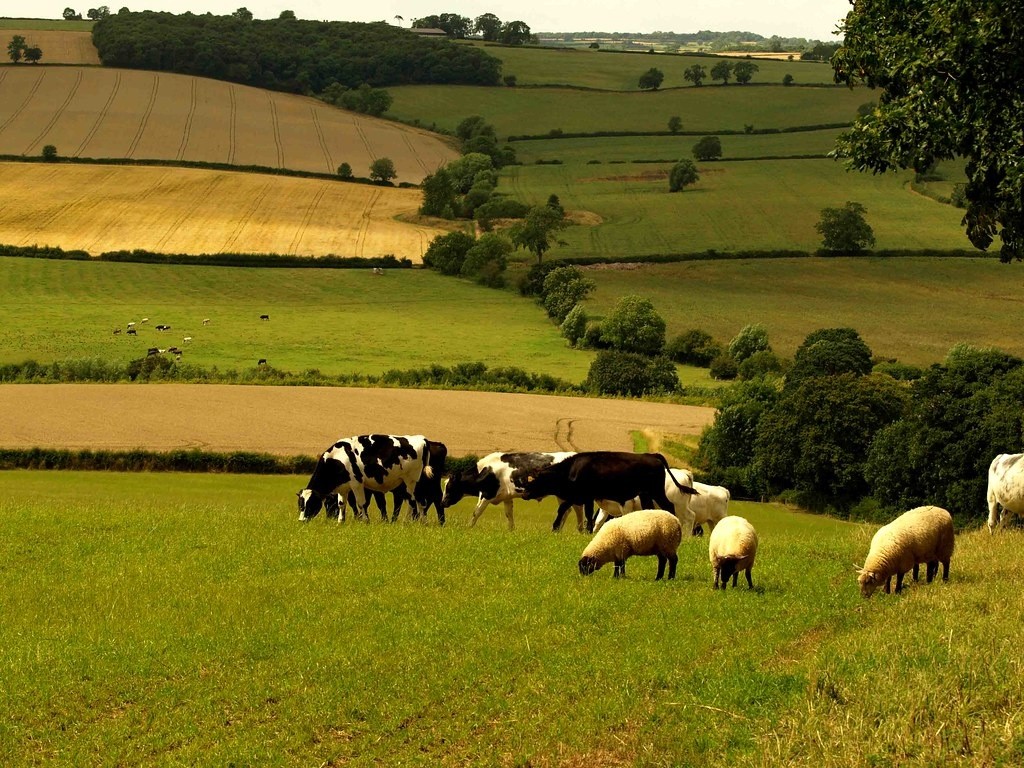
[708,515,758,590]
[986,451,1024,535]
[853,505,955,598]
[579,509,682,583]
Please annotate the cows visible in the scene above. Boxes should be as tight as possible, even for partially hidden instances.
[440,450,584,532]
[523,449,699,530]
[296,434,445,527]
[593,468,731,538]
